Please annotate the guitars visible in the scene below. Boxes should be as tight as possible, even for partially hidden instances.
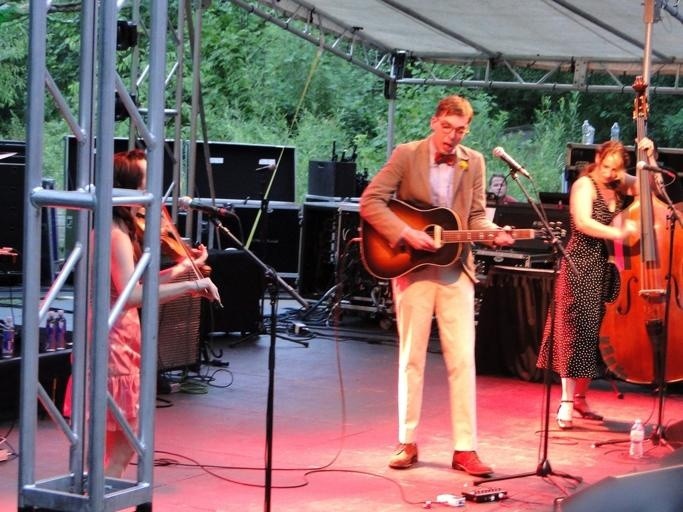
[361,198,567,280]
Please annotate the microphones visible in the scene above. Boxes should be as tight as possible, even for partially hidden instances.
[177,196,225,217]
[493,146,530,178]
[636,160,675,178]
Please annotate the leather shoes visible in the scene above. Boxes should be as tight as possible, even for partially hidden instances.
[388,444,417,469]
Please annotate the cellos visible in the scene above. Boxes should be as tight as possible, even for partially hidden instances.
[599,77,683,386]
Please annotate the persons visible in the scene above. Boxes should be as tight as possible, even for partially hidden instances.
[356,92,518,478]
[533,134,665,435]
[60,146,227,480]
[618,139,681,210]
[486,173,518,204]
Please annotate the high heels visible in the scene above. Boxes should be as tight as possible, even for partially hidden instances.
[555,396,602,430]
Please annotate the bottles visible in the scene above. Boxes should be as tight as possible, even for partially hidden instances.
[581,120,589,144]
[628,419,644,459]
[562,168,567,193]
[55,311,65,349]
[44,312,55,351]
[610,122,619,142]
[1,317,14,358]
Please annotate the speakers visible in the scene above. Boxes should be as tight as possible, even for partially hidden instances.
[308,160,356,197]
[137,259,204,373]
[204,250,261,336]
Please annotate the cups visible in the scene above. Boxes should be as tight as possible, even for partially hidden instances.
[589,127,595,144]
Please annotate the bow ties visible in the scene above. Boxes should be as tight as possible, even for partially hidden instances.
[433,150,457,166]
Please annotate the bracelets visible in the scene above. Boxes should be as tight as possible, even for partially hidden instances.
[187,278,201,299]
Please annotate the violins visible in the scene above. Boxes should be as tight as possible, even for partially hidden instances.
[133,202,212,276]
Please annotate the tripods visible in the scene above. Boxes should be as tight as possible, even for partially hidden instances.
[594,173,683,451]
[474,173,583,496]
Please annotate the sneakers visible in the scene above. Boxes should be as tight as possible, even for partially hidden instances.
[452,451,494,475]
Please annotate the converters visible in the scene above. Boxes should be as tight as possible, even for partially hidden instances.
[290,320,308,334]
